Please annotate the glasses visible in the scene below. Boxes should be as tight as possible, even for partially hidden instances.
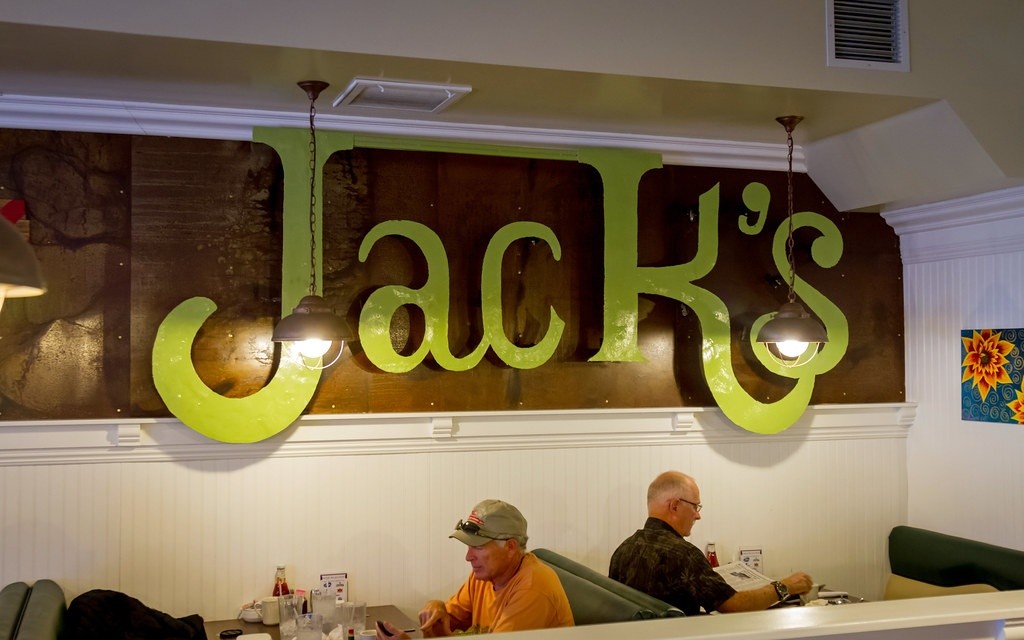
[454,519,514,541]
[679,498,702,512]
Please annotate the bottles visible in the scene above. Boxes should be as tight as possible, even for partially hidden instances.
[271,565,291,597]
[706,540,720,570]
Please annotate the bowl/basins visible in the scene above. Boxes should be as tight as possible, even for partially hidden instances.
[360,629,377,640]
[240,604,264,623]
[295,613,323,640]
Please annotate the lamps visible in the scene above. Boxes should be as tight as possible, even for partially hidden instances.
[269,80,355,373]
[757,114,829,368]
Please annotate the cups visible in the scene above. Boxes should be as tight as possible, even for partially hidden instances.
[310,587,337,634]
[277,594,303,640]
[799,582,819,606]
[254,596,281,625]
[342,602,367,640]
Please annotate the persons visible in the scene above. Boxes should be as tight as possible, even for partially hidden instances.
[375,499,574,640]
[607,471,813,617]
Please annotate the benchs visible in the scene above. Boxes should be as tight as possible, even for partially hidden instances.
[0,524,1024,640]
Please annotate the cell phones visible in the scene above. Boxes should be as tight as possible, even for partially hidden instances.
[377,620,393,637]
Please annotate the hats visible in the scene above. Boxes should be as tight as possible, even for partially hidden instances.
[448,499,528,547]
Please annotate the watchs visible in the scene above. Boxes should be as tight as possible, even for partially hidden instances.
[770,581,789,601]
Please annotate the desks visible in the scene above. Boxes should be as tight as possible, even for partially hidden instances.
[202,604,430,640]
